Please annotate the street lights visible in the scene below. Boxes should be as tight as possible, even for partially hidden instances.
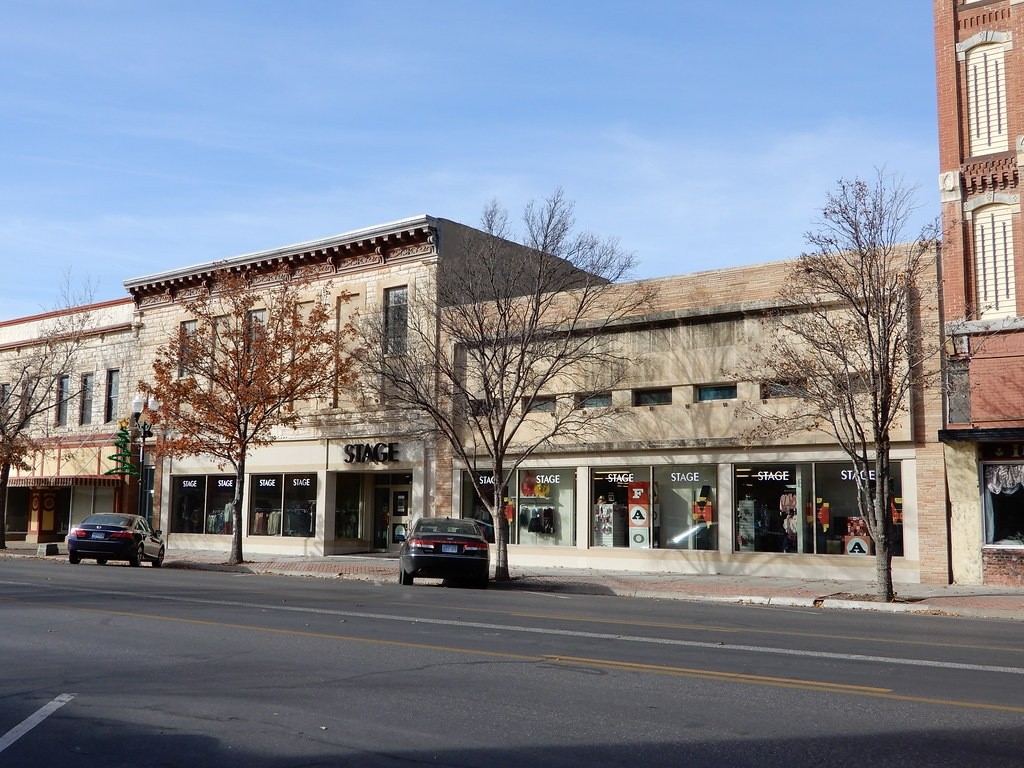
[132,390,159,515]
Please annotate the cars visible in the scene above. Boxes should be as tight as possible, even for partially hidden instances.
[394,516,492,588]
[640,522,791,555]
[65,510,166,567]
